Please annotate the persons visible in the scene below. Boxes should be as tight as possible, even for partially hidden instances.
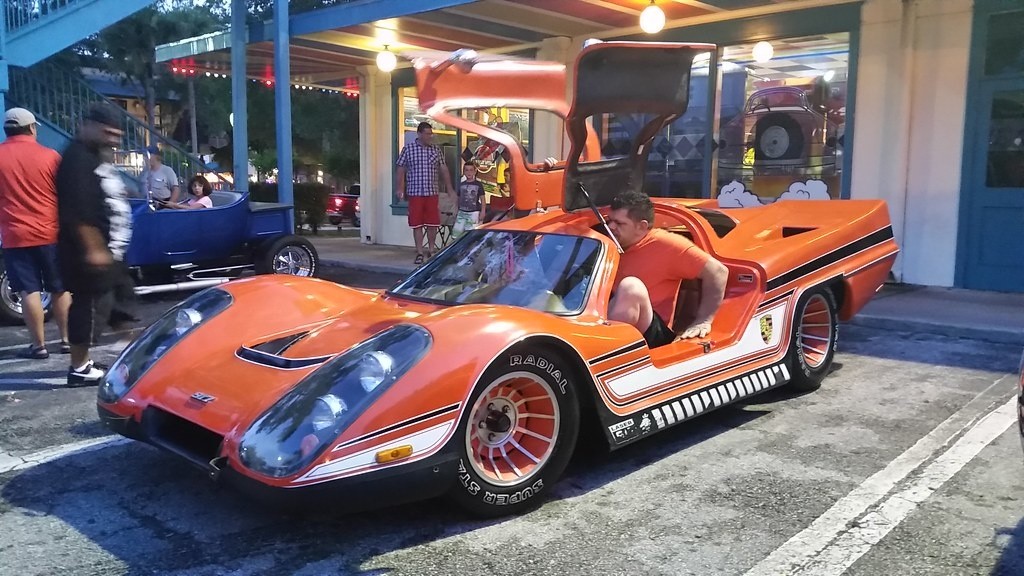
[395,122,511,264]
[607,188,729,349]
[0,106,71,360]
[141,145,179,211]
[161,175,214,210]
[54,103,135,389]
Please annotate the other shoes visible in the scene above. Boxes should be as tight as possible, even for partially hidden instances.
[428,251,436,258]
[414,254,424,264]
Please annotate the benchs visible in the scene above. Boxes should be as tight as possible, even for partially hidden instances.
[208,190,242,207]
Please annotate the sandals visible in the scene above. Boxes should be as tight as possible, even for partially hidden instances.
[17,343,49,359]
[60,341,71,353]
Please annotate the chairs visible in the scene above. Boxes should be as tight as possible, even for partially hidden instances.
[415,192,459,253]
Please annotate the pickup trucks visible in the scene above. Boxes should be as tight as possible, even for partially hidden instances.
[326,181,362,228]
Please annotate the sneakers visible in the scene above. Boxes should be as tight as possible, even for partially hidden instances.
[67,359,110,387]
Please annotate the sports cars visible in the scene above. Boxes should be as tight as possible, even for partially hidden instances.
[0,187,319,323]
[98,33,902,517]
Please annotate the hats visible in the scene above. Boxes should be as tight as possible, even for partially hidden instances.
[3,107,42,127]
[142,145,159,154]
[85,103,128,131]
